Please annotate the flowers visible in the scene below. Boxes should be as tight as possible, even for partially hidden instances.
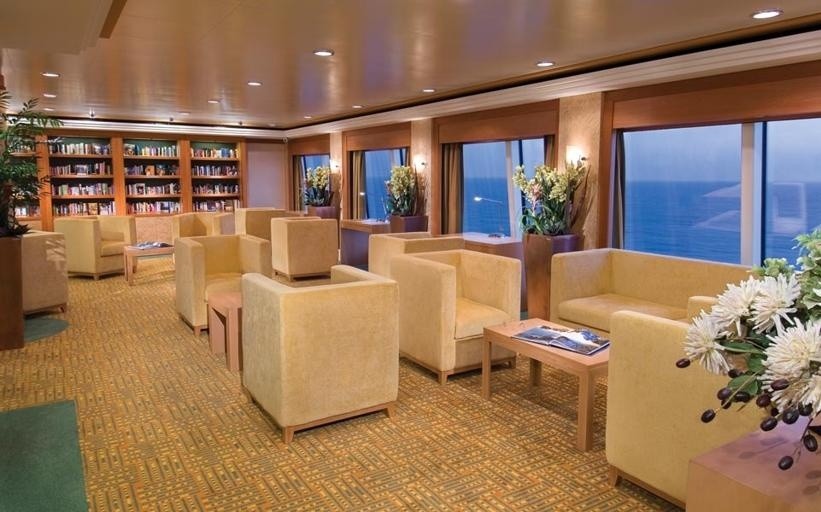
[378,164,430,218]
[293,162,335,207]
[665,219,820,471]
[513,155,599,241]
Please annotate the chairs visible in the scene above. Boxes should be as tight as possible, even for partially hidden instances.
[386,248,524,388]
[604,294,820,512]
[239,263,403,446]
[175,233,273,338]
[547,245,760,341]
[171,212,235,242]
[235,207,286,238]
[53,214,138,281]
[269,216,341,282]
[19,227,67,317]
[366,230,466,272]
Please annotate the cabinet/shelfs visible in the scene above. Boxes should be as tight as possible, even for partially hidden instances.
[1,125,248,241]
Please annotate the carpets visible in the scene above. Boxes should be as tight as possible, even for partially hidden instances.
[0,395,89,511]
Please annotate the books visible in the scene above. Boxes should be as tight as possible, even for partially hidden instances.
[133,241,174,250]
[15,143,240,217]
[511,325,609,355]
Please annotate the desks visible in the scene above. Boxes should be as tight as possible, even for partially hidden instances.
[122,247,174,286]
[478,315,611,453]
[206,292,242,372]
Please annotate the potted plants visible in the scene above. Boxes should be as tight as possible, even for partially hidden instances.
[0,83,66,351]
